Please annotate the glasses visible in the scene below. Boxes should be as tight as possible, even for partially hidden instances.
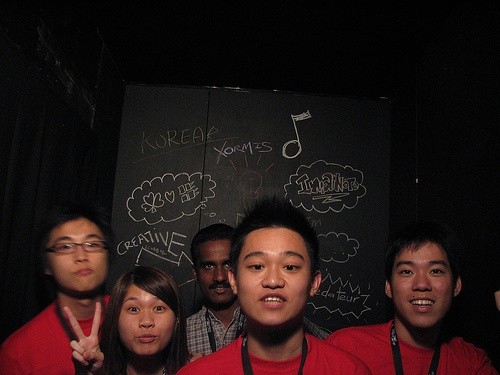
[47,239,109,254]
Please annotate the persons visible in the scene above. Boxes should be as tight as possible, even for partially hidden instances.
[173,189,374,375]
[162,221,338,362]
[321,222,499,375]
[0,204,138,375]
[61,264,208,375]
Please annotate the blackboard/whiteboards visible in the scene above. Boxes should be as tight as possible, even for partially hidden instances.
[112,85,392,332]
